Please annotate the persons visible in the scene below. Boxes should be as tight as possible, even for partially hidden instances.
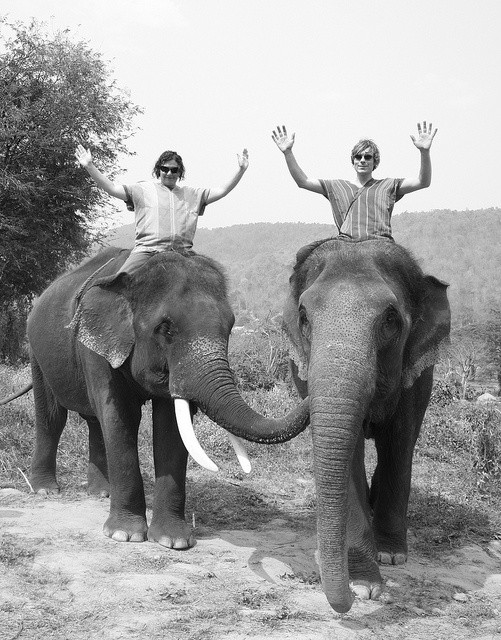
[271,119,439,241]
[73,142,251,277]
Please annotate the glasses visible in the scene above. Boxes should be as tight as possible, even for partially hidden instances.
[158,166,180,174]
[353,155,373,161]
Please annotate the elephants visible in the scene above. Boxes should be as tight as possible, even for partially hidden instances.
[24,246,313,551]
[278,237,452,615]
